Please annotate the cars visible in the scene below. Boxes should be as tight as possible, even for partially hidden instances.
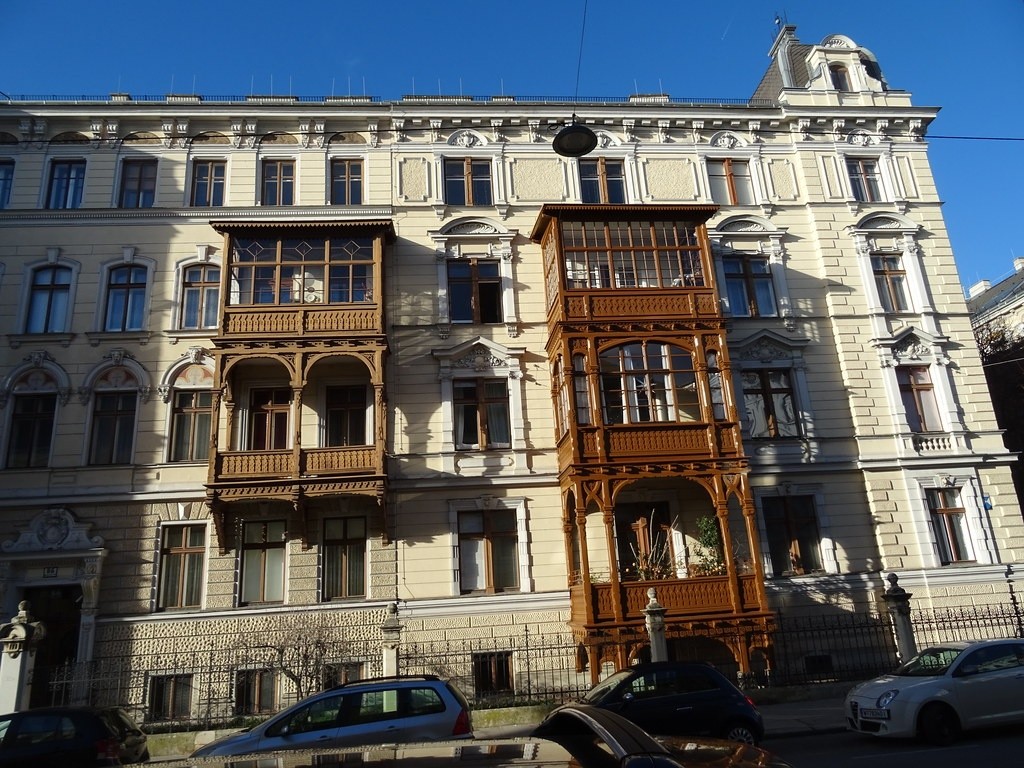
[0,705,151,768]
[844,636,1023,743]
[577,658,766,747]
[188,673,476,758]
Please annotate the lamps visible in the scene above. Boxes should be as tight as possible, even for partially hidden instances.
[549,111,598,160]
